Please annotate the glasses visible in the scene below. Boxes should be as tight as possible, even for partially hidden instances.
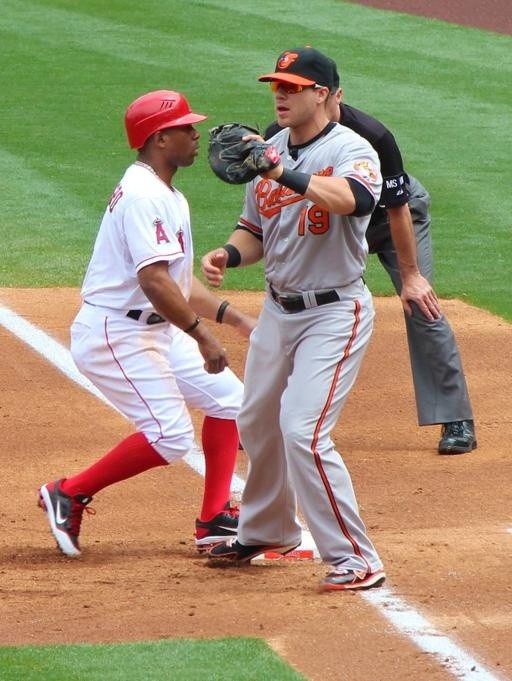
[271,80,313,93]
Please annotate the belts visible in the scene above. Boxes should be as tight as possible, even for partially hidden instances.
[270,287,337,313]
[127,309,165,325]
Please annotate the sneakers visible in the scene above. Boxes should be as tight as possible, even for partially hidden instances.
[316,567,387,591]
[194,503,302,564]
[438,421,477,454]
[37,478,94,560]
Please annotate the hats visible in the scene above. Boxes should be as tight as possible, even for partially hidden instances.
[259,46,336,88]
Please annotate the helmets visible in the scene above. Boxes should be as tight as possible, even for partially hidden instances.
[125,88,209,150]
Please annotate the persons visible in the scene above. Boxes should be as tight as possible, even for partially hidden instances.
[37,90,257,559]
[202,47,387,592]
[263,57,478,455]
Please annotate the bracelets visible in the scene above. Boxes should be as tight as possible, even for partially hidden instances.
[275,166,311,196]
[183,316,200,333]
[215,300,230,325]
[219,244,241,268]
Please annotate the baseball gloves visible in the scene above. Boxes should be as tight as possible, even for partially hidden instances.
[207,122,282,184]
